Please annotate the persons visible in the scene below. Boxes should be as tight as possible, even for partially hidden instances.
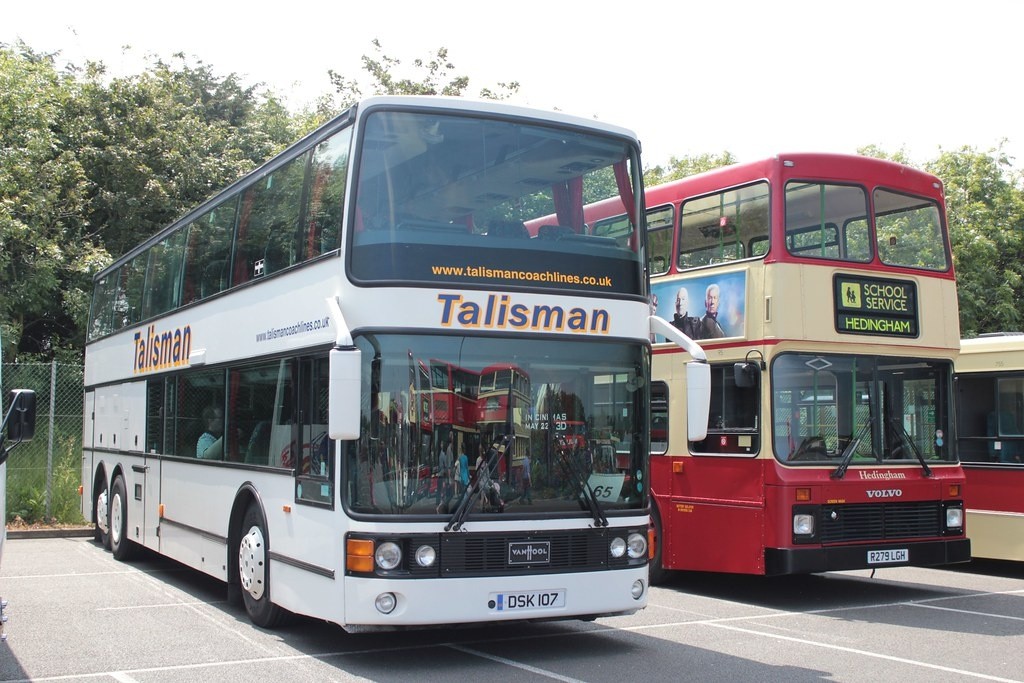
[195,404,243,459]
[650,294,658,343]
[519,450,533,506]
[666,283,726,341]
[435,430,495,514]
[560,438,591,501]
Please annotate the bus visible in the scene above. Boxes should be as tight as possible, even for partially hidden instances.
[523,153,972,586]
[781,332,1024,561]
[475,364,532,484]
[427,359,480,498]
[79,95,652,634]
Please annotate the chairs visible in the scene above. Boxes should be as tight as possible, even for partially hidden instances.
[775,422,793,460]
[355,182,577,242]
[94,205,342,339]
[245,421,272,465]
